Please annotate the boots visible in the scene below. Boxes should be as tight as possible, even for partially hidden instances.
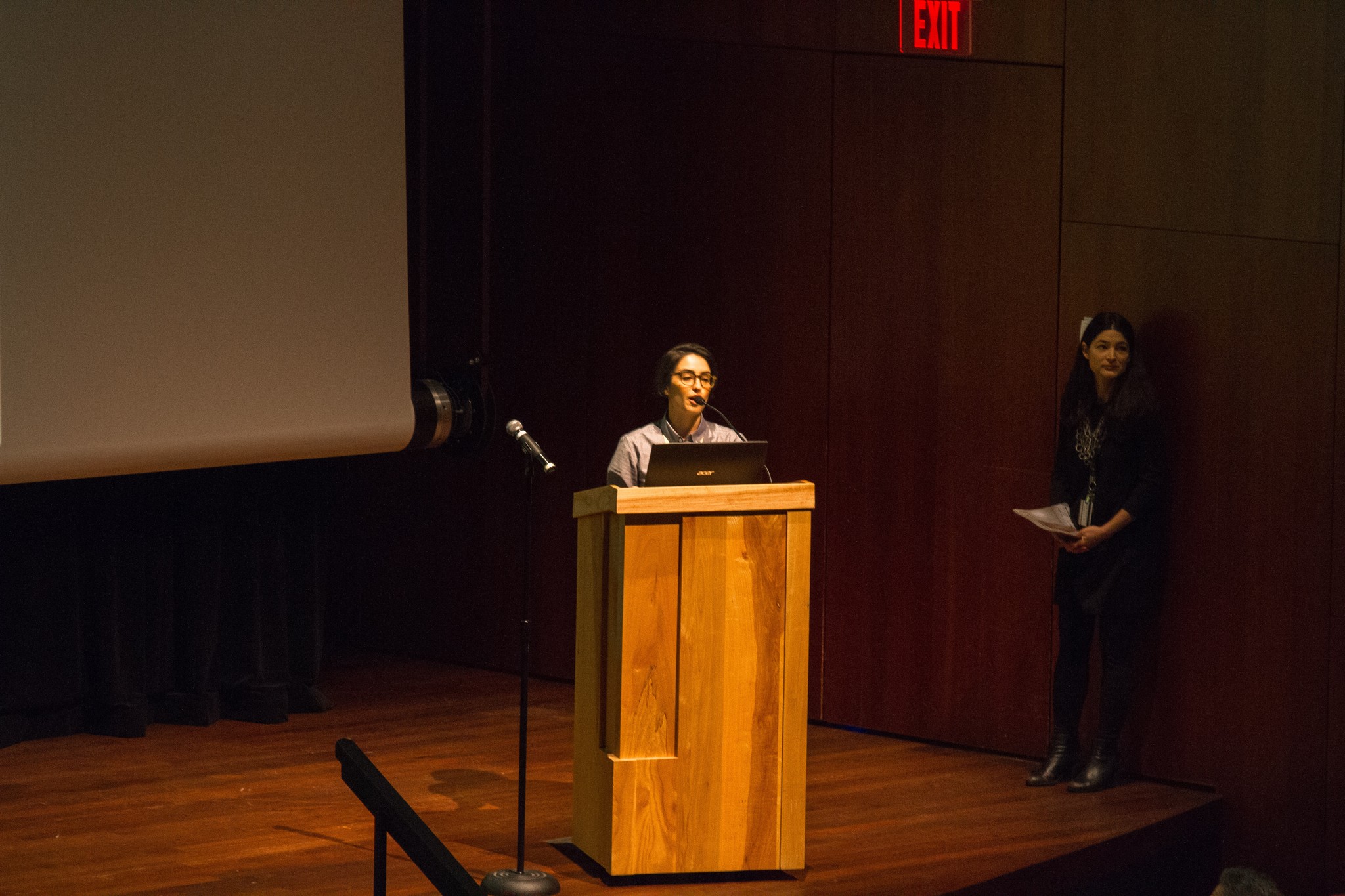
[1069,738,1119,792]
[1026,727,1079,787]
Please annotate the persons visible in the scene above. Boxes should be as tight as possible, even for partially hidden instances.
[1025,310,1168,796]
[606,344,750,487]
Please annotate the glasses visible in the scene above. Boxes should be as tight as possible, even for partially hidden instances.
[668,371,716,389]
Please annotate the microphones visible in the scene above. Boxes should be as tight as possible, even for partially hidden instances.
[505,420,557,473]
[694,395,773,484]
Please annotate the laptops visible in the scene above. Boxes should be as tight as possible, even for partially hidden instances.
[645,440,768,487]
